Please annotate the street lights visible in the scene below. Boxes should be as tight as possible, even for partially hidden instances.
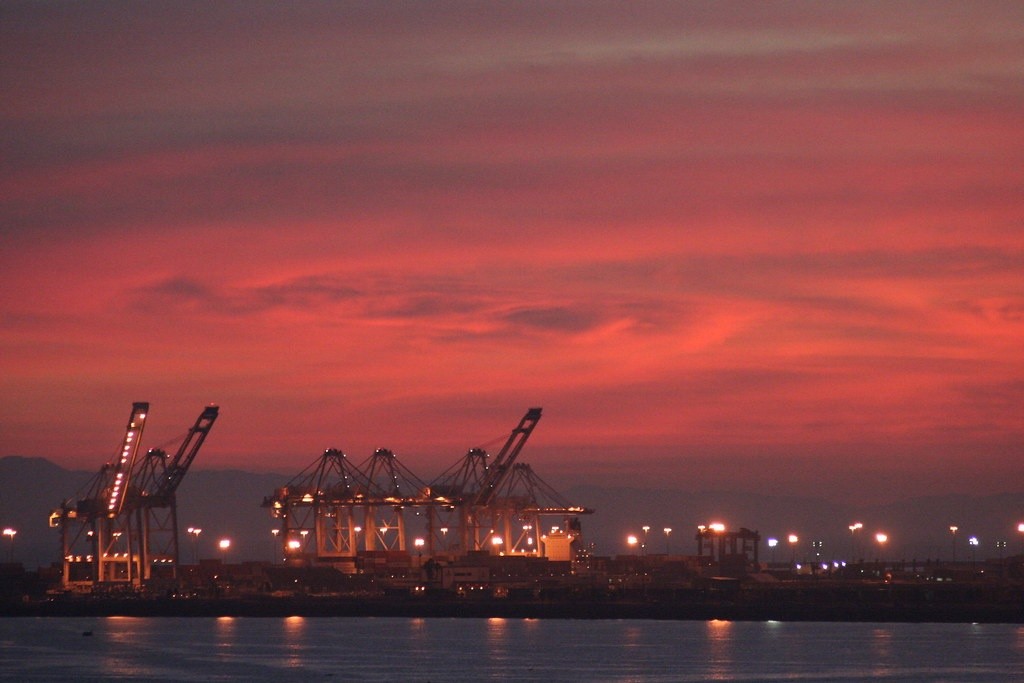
[715,524,725,576]
[950,526,959,570]
[812,541,822,583]
[997,540,1007,595]
[875,532,887,580]
[642,525,651,561]
[664,528,672,561]
[789,535,799,565]
[970,538,978,583]
[848,523,863,574]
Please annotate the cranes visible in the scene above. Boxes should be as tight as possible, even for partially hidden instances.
[42,400,221,601]
[257,406,598,597]
